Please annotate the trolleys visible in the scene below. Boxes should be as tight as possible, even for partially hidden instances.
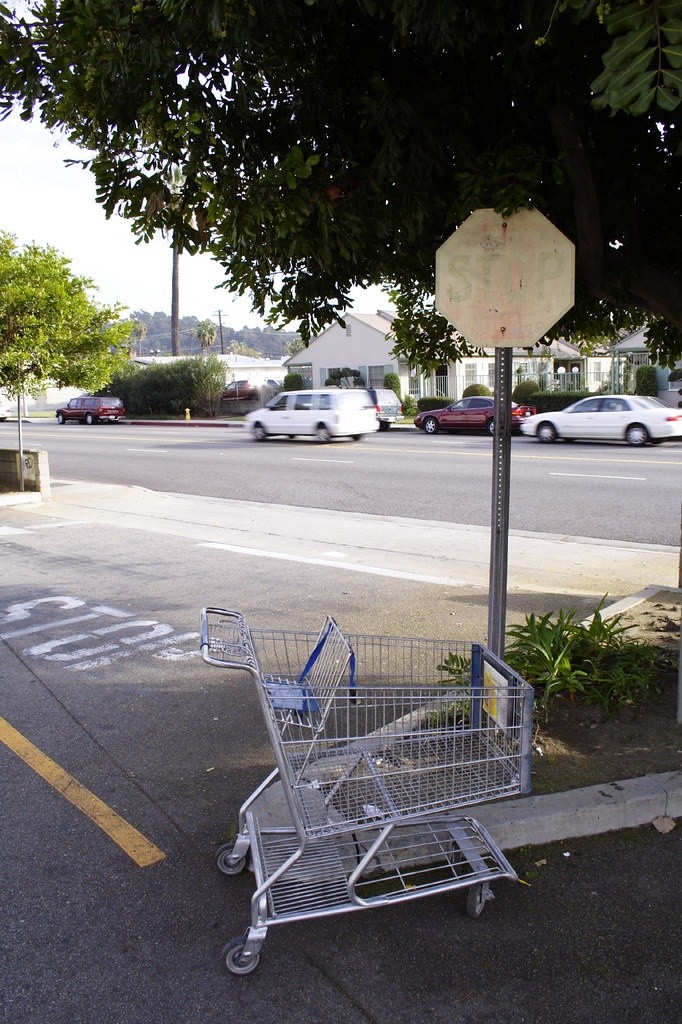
[198,605,536,975]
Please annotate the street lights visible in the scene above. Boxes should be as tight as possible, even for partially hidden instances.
[149,349,161,356]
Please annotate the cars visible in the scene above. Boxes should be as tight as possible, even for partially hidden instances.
[262,378,285,387]
[0,401,10,422]
[638,396,676,409]
[520,395,682,447]
[414,396,535,436]
[220,380,259,401]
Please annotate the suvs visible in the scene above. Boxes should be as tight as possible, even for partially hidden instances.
[242,389,380,443]
[374,389,405,431]
[55,396,125,425]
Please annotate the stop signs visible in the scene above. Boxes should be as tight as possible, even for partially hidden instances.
[434,207,576,348]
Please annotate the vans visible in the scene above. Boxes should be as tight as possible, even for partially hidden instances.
[345,387,380,440]
